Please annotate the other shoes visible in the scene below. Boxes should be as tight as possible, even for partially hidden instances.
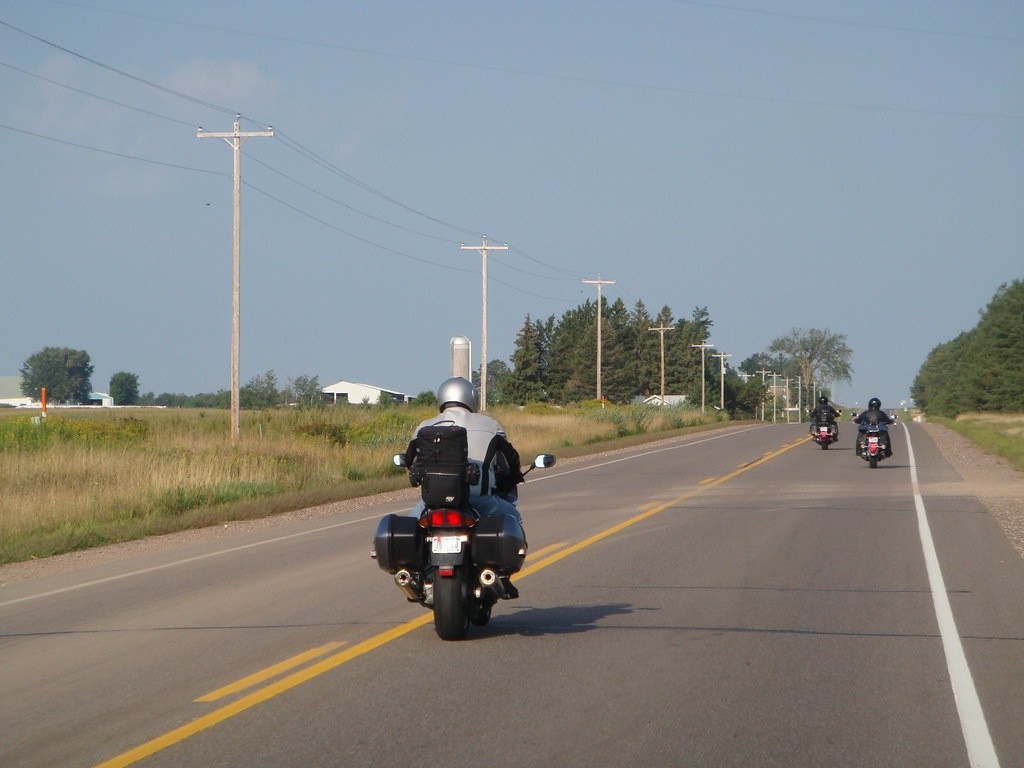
[500,576,519,599]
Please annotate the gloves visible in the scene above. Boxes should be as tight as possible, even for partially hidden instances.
[508,469,526,484]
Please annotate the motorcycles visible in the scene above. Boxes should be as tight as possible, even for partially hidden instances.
[806,408,844,449]
[851,413,898,468]
[373,454,556,640]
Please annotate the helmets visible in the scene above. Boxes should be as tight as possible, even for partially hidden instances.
[819,397,827,405]
[868,397,880,409]
[437,376,479,412]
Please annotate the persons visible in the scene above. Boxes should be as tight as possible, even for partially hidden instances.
[851,397,895,457]
[809,397,841,442]
[405,376,530,598]
